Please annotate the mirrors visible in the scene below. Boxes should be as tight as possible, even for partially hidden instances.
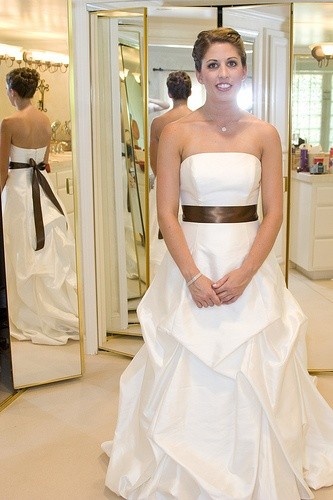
[88,3,333,372]
[91,7,150,312]
[0,0,84,390]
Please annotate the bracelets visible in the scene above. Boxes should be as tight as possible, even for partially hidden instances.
[186,272,203,288]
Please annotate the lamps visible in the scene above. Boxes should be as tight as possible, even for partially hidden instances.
[312,43,332,67]
[0,43,69,74]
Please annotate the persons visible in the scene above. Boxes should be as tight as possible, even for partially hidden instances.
[130,114,140,147]
[102,27,333,500]
[151,71,194,281]
[0,67,80,346]
[148,97,170,114]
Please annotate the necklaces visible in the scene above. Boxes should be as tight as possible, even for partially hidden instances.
[203,107,238,131]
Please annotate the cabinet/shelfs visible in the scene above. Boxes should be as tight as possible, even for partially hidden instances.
[48,170,74,232]
[291,172,331,282]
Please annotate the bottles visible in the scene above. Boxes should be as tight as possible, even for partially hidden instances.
[328,148,333,174]
[300,145,309,172]
